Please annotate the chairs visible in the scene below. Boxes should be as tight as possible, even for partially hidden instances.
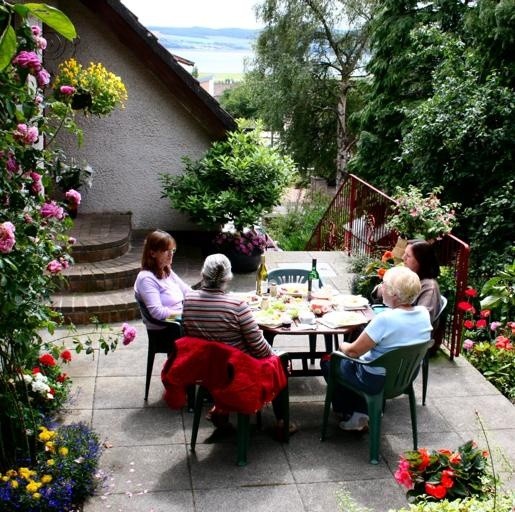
[173,335,290,467]
[134,292,195,413]
[422,295,448,405]
[319,339,435,465]
[268,269,316,364]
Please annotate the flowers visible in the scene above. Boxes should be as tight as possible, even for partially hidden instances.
[393,440,505,503]
[0,419,113,511]
[384,183,464,245]
[216,226,275,257]
[364,250,395,281]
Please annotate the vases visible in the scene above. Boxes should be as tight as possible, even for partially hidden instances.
[228,257,261,274]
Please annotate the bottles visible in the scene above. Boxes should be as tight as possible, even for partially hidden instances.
[256,255,268,296]
[308,259,319,301]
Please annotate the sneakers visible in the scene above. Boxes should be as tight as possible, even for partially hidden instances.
[274,419,297,435]
[339,417,369,431]
[206,405,229,427]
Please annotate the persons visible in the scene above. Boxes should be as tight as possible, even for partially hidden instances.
[134,229,213,406]
[320,267,434,432]
[348,240,441,342]
[182,254,300,436]
